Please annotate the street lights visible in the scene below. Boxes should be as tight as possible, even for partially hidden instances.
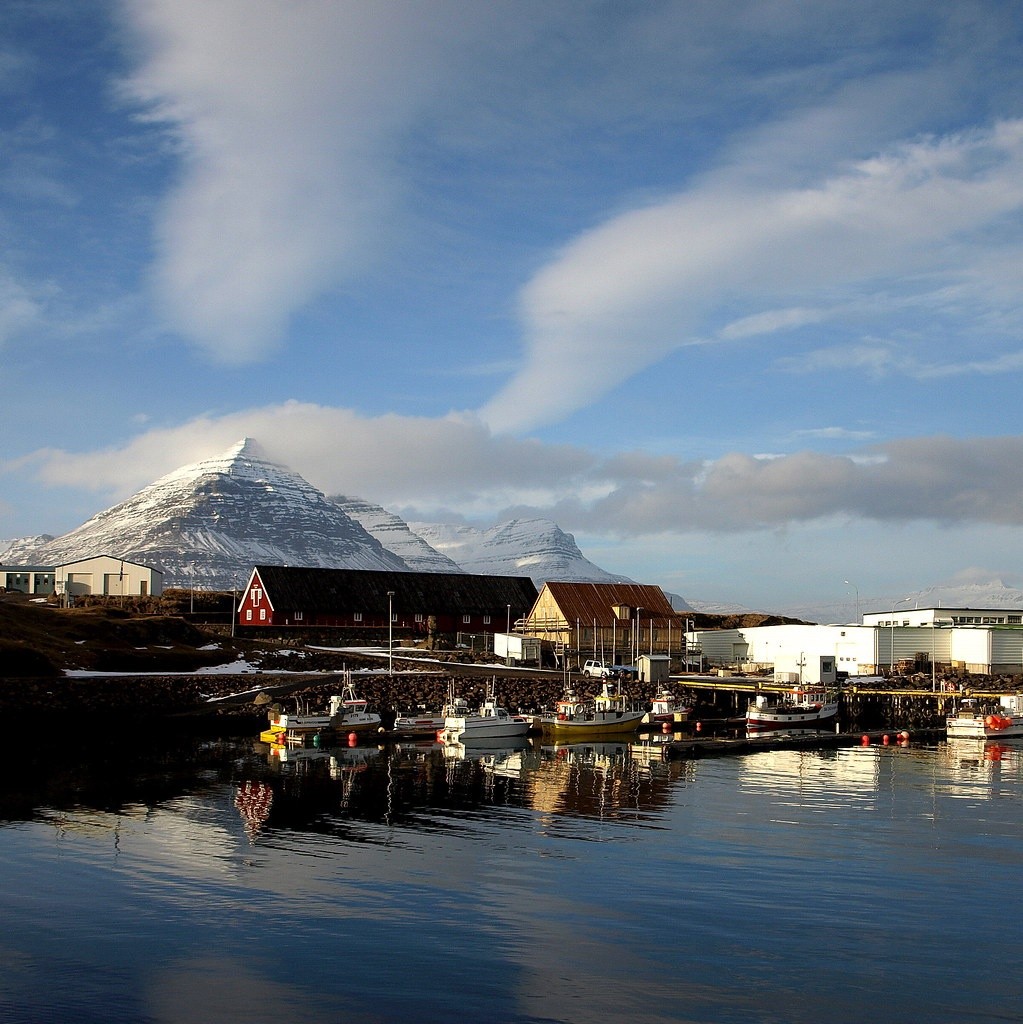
[387,591,395,676]
[637,607,644,669]
[231,574,238,637]
[191,562,195,613]
[470,635,476,649]
[507,604,510,667]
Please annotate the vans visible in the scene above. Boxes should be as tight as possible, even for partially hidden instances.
[582,659,614,679]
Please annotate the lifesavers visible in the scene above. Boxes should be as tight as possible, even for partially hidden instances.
[574,703,584,714]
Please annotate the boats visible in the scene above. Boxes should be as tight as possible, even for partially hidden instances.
[743,693,840,728]
[533,672,647,737]
[433,734,532,795]
[429,673,534,740]
[745,723,844,751]
[260,660,382,745]
[393,709,446,732]
[532,732,641,758]
